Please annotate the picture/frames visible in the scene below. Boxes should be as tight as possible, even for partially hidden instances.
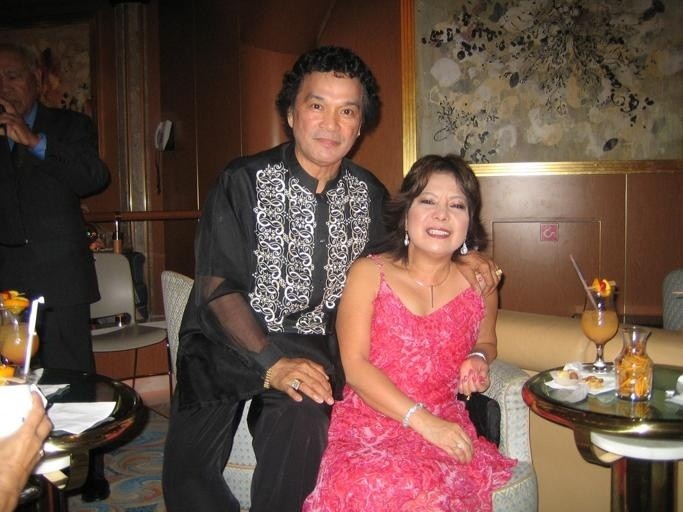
[401,0,683,179]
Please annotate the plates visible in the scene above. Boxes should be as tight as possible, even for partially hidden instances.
[580,374,615,396]
[544,380,578,391]
[546,387,589,404]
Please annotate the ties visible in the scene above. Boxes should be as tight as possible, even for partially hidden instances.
[11,141,26,167]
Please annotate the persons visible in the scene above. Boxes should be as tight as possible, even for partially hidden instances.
[300,153,512,511]
[0,389,54,511]
[0,43,120,373]
[160,43,399,511]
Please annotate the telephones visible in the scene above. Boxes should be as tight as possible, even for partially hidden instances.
[155,120,174,152]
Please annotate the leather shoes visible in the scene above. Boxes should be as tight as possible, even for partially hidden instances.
[82,476,109,502]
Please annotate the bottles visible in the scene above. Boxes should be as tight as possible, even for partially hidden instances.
[112,216,124,254]
[613,324,655,403]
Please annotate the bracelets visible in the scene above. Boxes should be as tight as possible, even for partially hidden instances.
[463,352,486,361]
[253,342,284,372]
[401,401,422,427]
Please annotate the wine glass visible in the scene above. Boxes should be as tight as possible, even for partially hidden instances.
[0,304,41,384]
[580,284,620,376]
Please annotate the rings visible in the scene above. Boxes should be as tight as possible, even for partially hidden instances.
[38,449,45,456]
[291,378,301,389]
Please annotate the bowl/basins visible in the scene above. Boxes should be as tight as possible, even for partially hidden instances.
[549,369,585,386]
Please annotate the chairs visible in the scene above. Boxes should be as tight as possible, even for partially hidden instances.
[90,253,174,421]
[161,270,538,512]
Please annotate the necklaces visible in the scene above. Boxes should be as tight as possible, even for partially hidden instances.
[402,254,451,308]
